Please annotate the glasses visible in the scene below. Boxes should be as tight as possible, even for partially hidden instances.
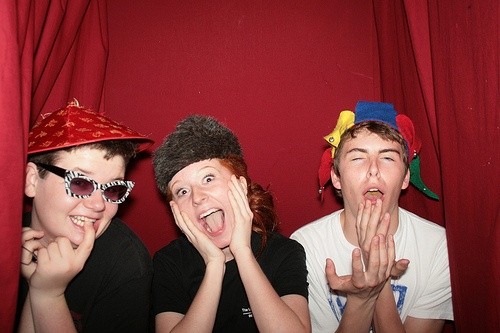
[25,163,135,204]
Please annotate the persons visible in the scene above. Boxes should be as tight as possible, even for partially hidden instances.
[151,112,311,333]
[17,97,153,333]
[288,100,454,333]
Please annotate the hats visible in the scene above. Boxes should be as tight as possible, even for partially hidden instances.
[27,96,156,163]
[154,113,249,193]
[317,101,440,203]
